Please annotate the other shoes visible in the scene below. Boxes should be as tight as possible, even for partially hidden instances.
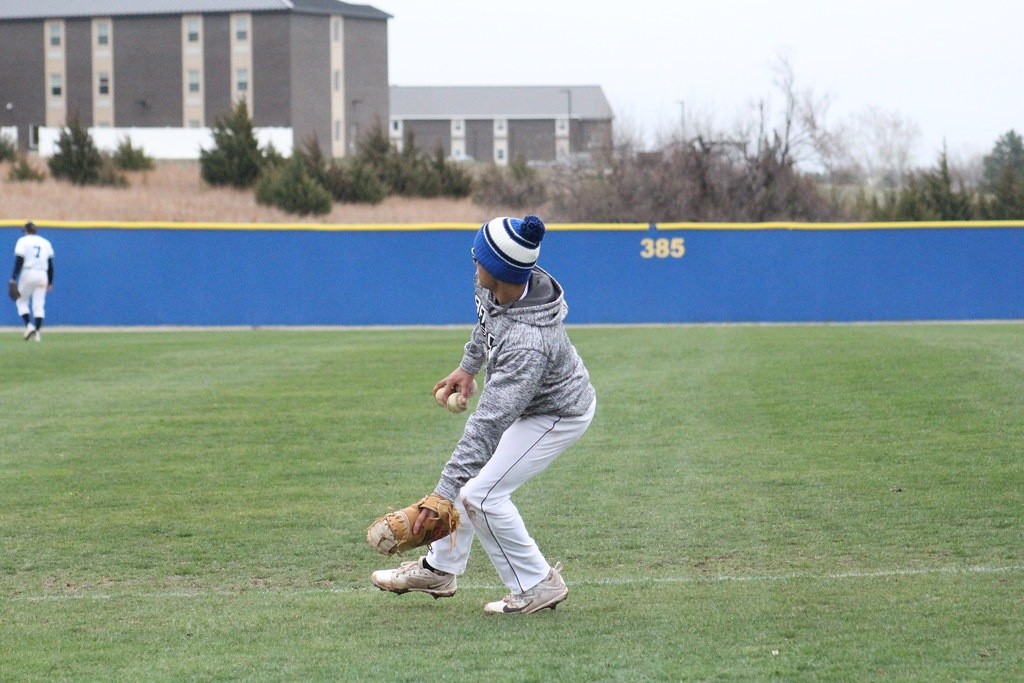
[24,323,41,342]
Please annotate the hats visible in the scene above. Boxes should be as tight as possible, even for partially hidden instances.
[473,215,545,284]
[22,223,38,231]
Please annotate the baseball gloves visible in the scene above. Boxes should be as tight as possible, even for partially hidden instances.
[9,283,21,301]
[368,494,460,557]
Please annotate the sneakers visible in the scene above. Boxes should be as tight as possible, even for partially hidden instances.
[372,556,458,600]
[485,569,569,614]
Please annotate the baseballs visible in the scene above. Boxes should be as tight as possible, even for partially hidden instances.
[434,387,454,407]
[447,393,469,413]
[456,378,478,397]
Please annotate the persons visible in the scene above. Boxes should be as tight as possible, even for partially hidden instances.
[8,223,55,341]
[367,214,596,616]
